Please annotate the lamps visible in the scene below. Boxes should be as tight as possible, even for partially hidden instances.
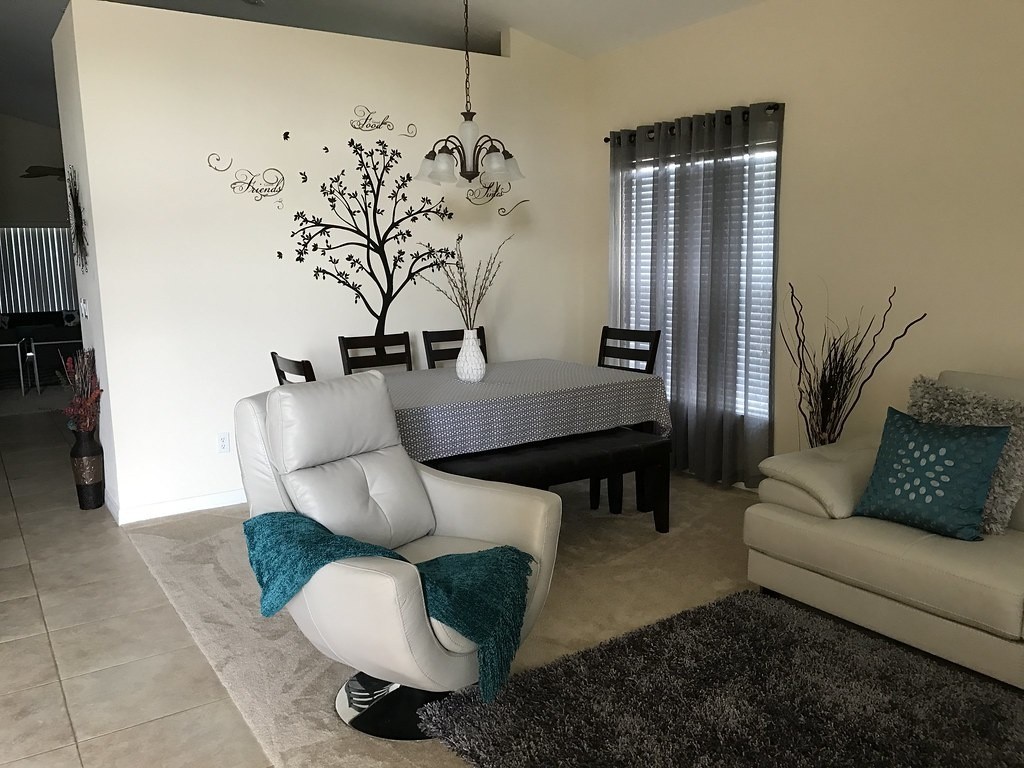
[413,0,524,186]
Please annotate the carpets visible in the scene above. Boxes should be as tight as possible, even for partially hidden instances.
[419,589,1024,768]
[125,474,761,768]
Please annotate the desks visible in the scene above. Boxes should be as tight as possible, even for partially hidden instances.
[383,359,672,461]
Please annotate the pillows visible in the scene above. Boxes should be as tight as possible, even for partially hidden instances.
[905,374,1024,537]
[853,407,1012,541]
[63,309,80,327]
[0,312,10,329]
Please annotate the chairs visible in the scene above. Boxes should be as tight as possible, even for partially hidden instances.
[0,336,31,397]
[271,351,316,386]
[30,327,84,396]
[232,371,562,743]
[588,325,661,510]
[338,331,413,377]
[422,326,489,370]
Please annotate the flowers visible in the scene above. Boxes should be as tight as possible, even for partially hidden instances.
[56,347,106,431]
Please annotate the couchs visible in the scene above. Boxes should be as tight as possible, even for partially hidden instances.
[0,310,78,352]
[743,370,1024,692]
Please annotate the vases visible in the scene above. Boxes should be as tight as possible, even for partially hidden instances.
[456,328,487,381]
[71,428,106,510]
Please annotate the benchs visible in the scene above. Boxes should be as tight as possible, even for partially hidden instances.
[420,428,672,533]
